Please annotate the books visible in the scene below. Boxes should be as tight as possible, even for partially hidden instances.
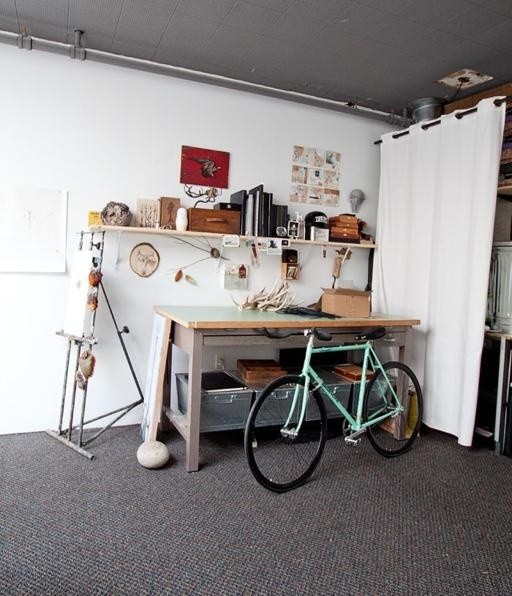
[230,184,290,238]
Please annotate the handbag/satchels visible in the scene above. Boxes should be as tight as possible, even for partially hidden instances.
[376,385,419,439]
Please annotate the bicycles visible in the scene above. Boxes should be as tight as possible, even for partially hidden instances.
[244,325,423,493]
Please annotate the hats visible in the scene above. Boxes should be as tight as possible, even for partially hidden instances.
[304,212,330,229]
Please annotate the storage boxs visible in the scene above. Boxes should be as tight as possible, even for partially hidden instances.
[187,208,241,235]
[176,370,395,432]
[321,287,370,318]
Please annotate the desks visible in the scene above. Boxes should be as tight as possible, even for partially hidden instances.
[475,331,512,456]
[148,306,421,471]
